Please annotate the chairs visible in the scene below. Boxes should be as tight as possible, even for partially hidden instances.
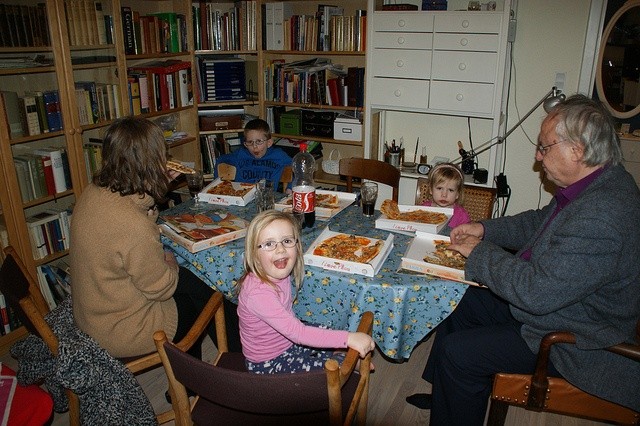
[485,331,639,426]
[339,157,400,216]
[1,245,177,424]
[152,290,374,426]
[217,162,293,195]
[414,176,497,222]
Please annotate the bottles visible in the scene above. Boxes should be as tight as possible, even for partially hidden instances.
[292,143,315,229]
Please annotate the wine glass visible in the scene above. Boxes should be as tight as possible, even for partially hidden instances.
[185,172,207,210]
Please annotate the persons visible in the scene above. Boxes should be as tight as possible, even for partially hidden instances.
[404,93,640,426]
[67,116,241,404]
[214,119,294,196]
[415,162,471,229]
[234,209,375,376]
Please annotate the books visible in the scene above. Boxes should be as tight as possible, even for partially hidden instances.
[83,136,104,184]
[194,0,258,174]
[0,1,77,337]
[66,2,194,127]
[261,1,364,105]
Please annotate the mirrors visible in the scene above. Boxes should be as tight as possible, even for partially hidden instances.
[595,0,640,119]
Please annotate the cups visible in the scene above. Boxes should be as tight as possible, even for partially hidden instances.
[360,182,379,218]
[255,189,275,212]
[461,158,478,174]
[256,179,274,191]
[389,151,400,167]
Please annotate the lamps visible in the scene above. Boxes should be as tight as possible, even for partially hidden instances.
[449,86,566,166]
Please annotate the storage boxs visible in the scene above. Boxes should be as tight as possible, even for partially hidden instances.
[279,108,315,137]
[333,117,363,142]
[197,108,246,132]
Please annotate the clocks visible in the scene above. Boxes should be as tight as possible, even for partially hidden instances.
[417,163,432,175]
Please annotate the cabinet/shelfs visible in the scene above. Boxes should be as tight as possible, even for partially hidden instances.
[188,0,261,183]
[120,1,201,172]
[617,132,640,190]
[261,0,369,193]
[364,0,504,218]
[0,1,130,313]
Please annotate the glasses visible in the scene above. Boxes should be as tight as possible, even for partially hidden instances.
[256,235,297,252]
[242,137,267,147]
[538,139,565,153]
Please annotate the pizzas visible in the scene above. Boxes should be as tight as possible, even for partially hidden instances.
[163,159,196,176]
[218,162,237,182]
[205,180,256,197]
[423,238,468,271]
[312,232,384,264]
[380,199,449,226]
[286,192,344,213]
